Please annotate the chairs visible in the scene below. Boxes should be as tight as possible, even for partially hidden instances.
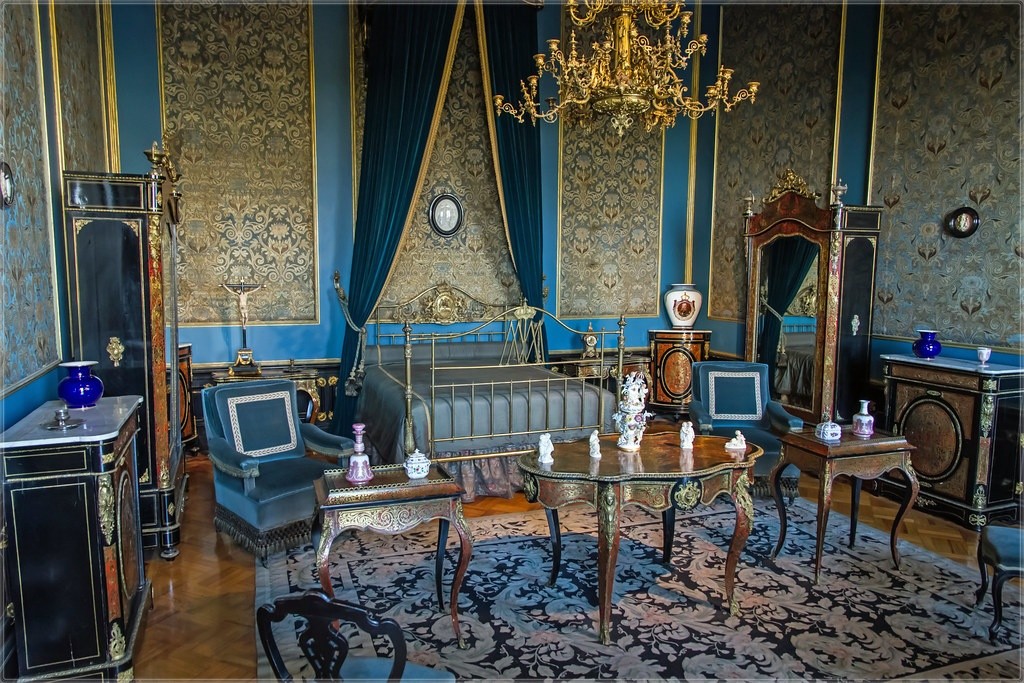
[972,526,1024,643]
[256,592,457,683]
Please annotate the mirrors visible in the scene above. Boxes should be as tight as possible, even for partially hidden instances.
[743,169,845,430]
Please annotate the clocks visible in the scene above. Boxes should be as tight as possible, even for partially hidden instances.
[580,322,601,359]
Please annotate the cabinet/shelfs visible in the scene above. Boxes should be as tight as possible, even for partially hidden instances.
[0,396,155,683]
[872,353,1024,534]
[177,343,200,457]
[648,330,713,414]
[59,171,190,564]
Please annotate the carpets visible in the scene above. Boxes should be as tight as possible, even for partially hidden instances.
[255,489,1024,683]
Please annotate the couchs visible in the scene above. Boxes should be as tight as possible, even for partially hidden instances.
[687,362,804,508]
[200,379,355,567]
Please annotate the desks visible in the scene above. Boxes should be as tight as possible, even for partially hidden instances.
[769,426,920,586]
[515,432,770,650]
[559,355,652,408]
[309,460,475,651]
[209,369,321,426]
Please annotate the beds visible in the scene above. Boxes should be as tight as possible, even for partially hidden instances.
[356,280,627,465]
[758,313,818,399]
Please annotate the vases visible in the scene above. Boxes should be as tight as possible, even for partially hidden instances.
[663,282,703,331]
[58,361,105,411]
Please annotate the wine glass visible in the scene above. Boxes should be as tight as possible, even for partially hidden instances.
[977,347,991,367]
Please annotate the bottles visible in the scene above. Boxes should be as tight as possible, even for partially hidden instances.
[851,400,874,438]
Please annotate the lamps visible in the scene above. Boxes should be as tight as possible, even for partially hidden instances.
[490,0,760,139]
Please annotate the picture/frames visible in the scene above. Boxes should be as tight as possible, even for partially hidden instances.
[427,193,464,239]
